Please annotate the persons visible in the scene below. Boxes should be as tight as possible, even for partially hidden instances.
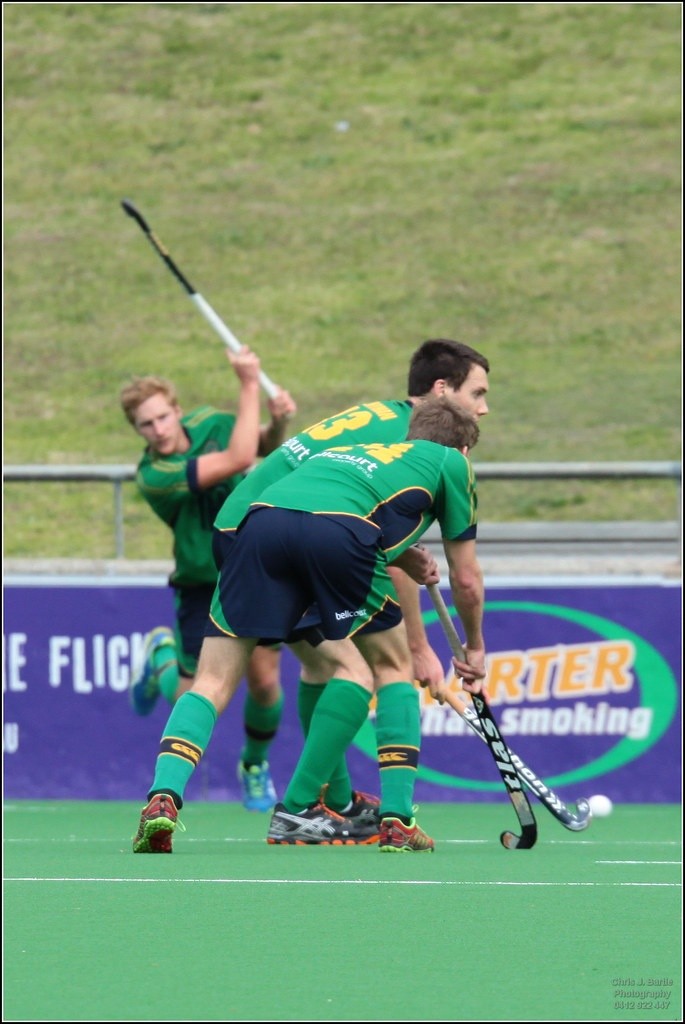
[127,394,488,854]
[116,342,300,814]
[209,338,493,847]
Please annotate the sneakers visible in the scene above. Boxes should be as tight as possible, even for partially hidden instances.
[319,791,378,816]
[265,802,382,846]
[131,795,178,852]
[320,783,382,805]
[238,757,277,812]
[130,626,176,716]
[378,816,435,854]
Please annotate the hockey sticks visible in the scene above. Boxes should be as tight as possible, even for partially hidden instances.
[440,686,589,832]
[413,542,538,849]
[121,201,278,400]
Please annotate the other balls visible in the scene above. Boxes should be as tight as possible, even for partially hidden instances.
[588,794,612,817]
